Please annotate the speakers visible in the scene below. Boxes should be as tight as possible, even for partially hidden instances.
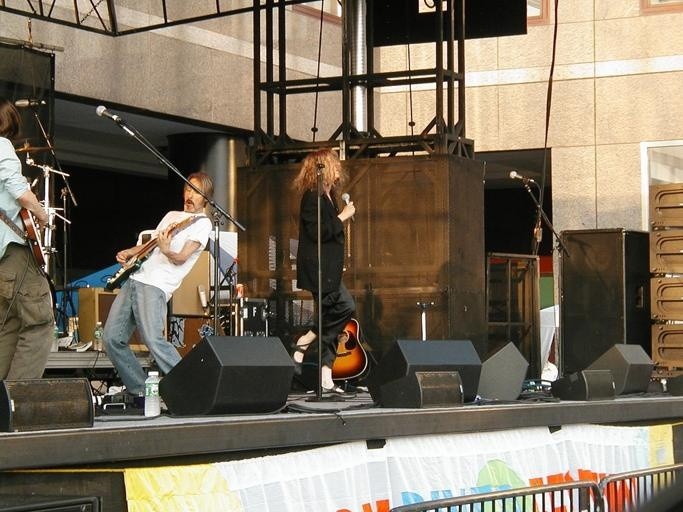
[586,344,655,396]
[476,341,530,402]
[159,336,296,416]
[366,340,482,403]
[1,378,94,432]
[563,228,650,376]
[374,371,464,408]
[551,370,616,401]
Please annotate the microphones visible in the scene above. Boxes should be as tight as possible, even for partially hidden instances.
[198,284,208,308]
[96,106,121,122]
[509,171,535,183]
[15,98,45,108]
[342,193,355,222]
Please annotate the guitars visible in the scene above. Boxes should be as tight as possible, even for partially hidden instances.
[331,318,370,384]
[106,214,195,291]
[20,207,49,268]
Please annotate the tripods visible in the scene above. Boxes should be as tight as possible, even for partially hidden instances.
[22,110,76,338]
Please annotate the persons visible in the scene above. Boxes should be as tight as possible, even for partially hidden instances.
[0,97,55,379]
[100,172,214,395]
[288,147,358,399]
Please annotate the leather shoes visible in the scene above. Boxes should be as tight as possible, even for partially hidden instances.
[290,333,307,375]
[321,384,357,399]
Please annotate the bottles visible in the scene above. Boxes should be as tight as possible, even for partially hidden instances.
[144,370,161,417]
[50,321,59,352]
[93,320,103,352]
